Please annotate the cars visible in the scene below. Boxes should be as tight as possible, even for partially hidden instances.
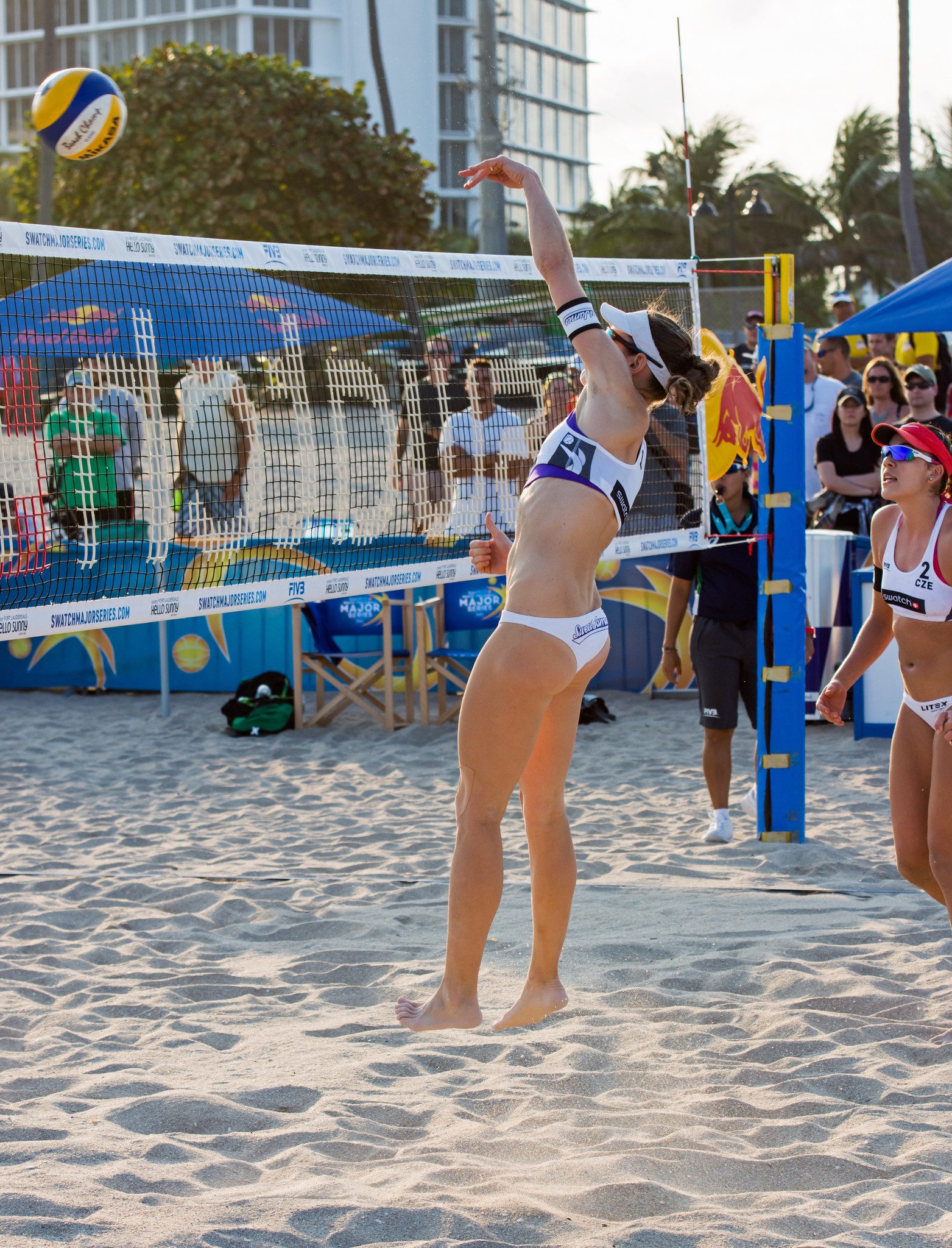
[377,327,575,362]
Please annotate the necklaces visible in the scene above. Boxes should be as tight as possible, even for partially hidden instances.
[868,402,889,422]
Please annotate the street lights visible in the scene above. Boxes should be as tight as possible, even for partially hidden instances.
[691,181,775,345]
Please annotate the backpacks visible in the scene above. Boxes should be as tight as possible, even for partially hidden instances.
[908,331,952,416]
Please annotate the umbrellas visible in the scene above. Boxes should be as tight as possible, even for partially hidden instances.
[817,257,952,340]
[0,261,412,719]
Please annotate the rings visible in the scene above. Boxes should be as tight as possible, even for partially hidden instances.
[809,656,812,660]
[489,167,494,174]
[950,741,952,745]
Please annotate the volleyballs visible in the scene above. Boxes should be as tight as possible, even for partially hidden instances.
[31,68,128,160]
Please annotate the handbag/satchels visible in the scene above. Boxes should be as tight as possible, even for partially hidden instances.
[220,671,295,733]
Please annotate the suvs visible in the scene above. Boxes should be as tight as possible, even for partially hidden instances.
[218,356,267,374]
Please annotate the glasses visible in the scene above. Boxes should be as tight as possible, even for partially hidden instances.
[745,323,757,329]
[429,350,446,356]
[866,376,891,383]
[904,381,931,390]
[817,348,837,357]
[881,445,940,466]
[605,326,664,370]
[725,463,745,474]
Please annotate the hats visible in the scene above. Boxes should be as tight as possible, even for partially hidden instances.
[871,422,952,479]
[904,364,936,387]
[804,341,814,354]
[746,310,764,319]
[832,290,857,307]
[600,302,675,397]
[64,369,92,388]
[837,386,867,407]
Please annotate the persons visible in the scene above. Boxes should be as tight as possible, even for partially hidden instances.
[76,357,146,522]
[660,454,814,842]
[393,153,720,1033]
[174,359,256,539]
[43,368,127,540]
[389,290,952,541]
[816,423,952,927]
[0,483,48,554]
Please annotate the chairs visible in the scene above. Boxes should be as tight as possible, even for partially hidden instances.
[292,586,414,734]
[414,574,508,726]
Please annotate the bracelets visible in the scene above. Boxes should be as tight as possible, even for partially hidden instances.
[805,627,816,640]
[661,646,677,653]
[871,488,875,497]
[233,469,244,478]
[556,297,604,342]
[870,566,882,592]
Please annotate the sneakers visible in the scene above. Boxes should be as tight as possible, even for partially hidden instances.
[702,810,733,843]
